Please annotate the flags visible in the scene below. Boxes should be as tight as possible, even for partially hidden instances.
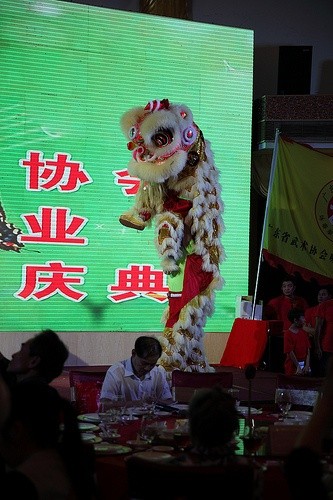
[263,133,333,284]
[263,134,333,279]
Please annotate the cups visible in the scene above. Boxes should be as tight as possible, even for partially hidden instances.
[177,419,189,432]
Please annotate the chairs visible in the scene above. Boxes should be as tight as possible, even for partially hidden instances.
[69,370,107,414]
[124,455,291,500]
[171,369,233,404]
[275,389,319,411]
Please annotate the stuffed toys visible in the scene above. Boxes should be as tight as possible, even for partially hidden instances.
[119,98,226,371]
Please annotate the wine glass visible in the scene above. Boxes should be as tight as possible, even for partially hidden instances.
[278,391,292,420]
[99,395,158,443]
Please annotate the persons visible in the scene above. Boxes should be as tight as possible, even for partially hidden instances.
[265,279,333,376]
[119,151,200,248]
[0,330,97,500]
[100,336,173,412]
[143,387,263,500]
[284,387,333,500]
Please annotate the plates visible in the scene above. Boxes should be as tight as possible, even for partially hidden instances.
[77,405,190,460]
[288,410,313,418]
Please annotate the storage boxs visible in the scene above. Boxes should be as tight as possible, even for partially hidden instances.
[236,296,263,320]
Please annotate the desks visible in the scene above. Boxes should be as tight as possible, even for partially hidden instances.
[81,408,315,500]
